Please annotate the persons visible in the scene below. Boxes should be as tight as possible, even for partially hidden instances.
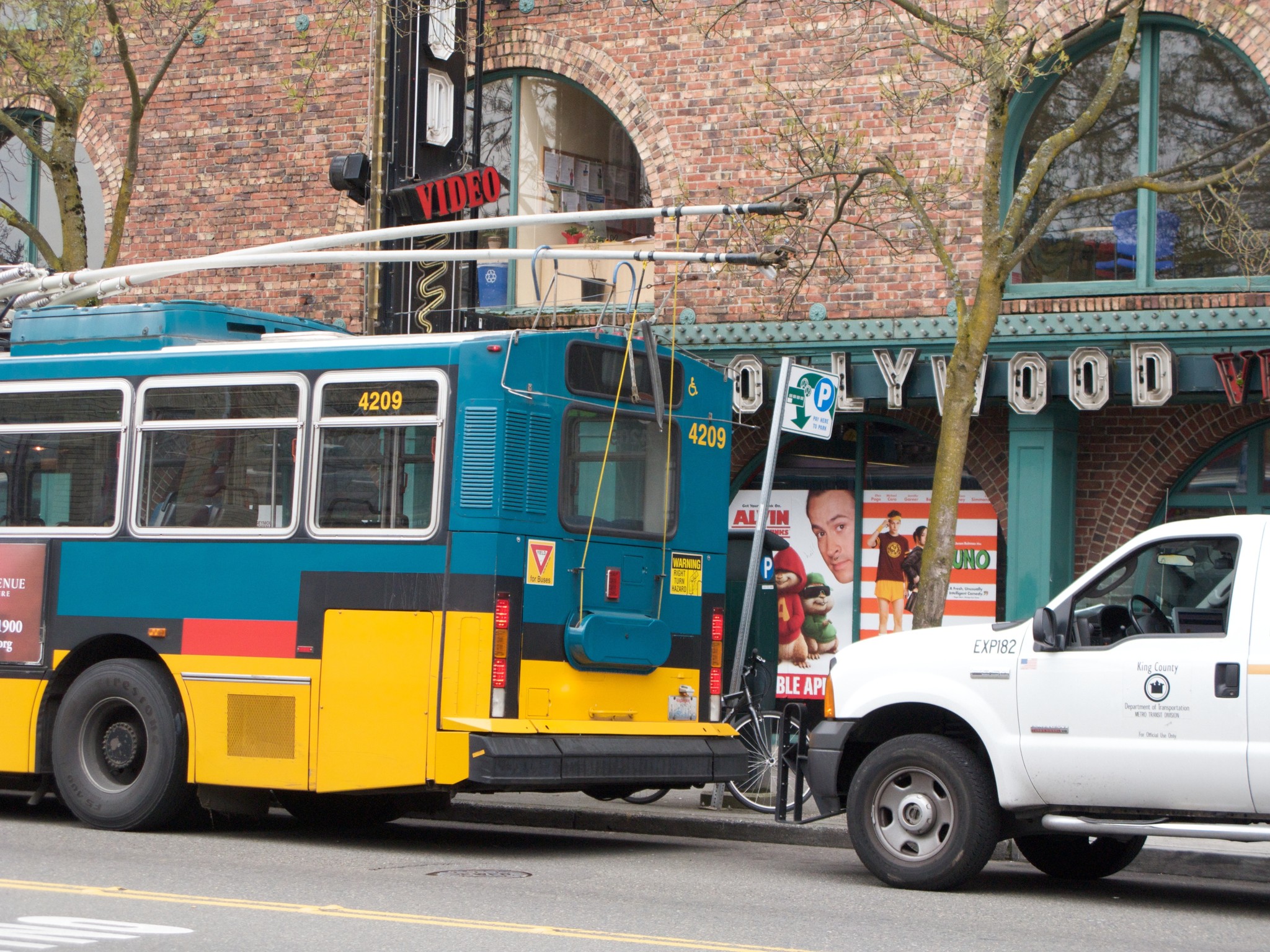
[899,525,929,614]
[866,512,909,636]
[807,487,855,582]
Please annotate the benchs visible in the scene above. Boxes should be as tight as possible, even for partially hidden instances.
[319,497,410,529]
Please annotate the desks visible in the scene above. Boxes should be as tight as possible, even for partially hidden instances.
[1065,226,1135,282]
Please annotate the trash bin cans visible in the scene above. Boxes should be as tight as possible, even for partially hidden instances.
[477,262,508,307]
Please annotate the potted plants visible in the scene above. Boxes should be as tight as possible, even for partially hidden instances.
[580,225,607,301]
[561,226,585,244]
[482,228,508,248]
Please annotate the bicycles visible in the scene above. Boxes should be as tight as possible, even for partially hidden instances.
[616,645,815,815]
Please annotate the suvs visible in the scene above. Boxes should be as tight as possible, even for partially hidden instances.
[803,511,1270,895]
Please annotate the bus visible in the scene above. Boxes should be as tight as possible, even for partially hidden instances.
[0,191,812,835]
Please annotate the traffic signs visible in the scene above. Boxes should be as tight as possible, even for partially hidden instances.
[781,363,841,440]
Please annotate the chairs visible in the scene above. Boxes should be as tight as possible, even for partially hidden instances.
[160,489,212,527]
[212,485,260,527]
[1094,207,1181,279]
